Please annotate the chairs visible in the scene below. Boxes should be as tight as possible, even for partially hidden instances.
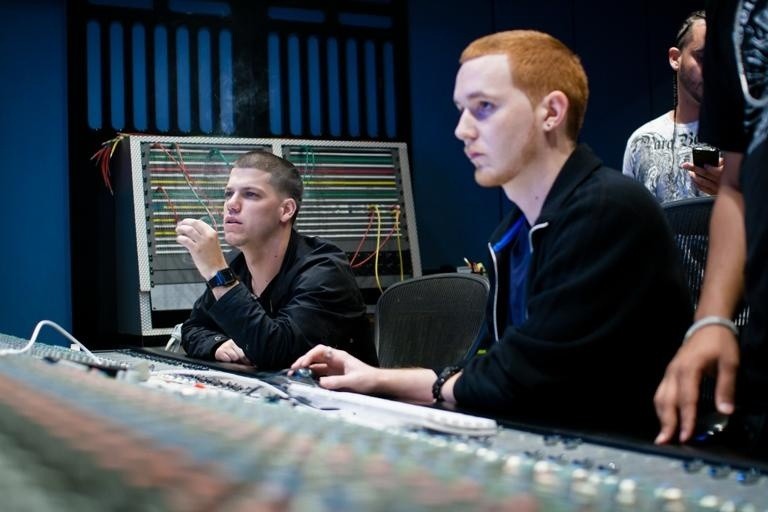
[664,196,752,332]
[373,272,490,377]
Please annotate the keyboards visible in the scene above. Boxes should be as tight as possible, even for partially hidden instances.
[286,383,497,435]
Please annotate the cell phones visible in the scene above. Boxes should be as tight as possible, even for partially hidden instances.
[691,146,718,168]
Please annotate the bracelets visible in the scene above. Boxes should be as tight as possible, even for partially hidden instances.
[684,315,738,346]
[433,365,460,403]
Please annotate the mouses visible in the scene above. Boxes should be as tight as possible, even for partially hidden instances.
[278,368,327,386]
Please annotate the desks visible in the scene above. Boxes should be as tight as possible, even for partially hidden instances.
[0,331,766,512]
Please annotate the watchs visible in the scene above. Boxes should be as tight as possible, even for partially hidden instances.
[206,268,237,289]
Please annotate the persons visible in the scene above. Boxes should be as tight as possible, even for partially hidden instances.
[289,29,696,443]
[621,12,723,204]
[175,152,379,369]
[653,1,768,445]
